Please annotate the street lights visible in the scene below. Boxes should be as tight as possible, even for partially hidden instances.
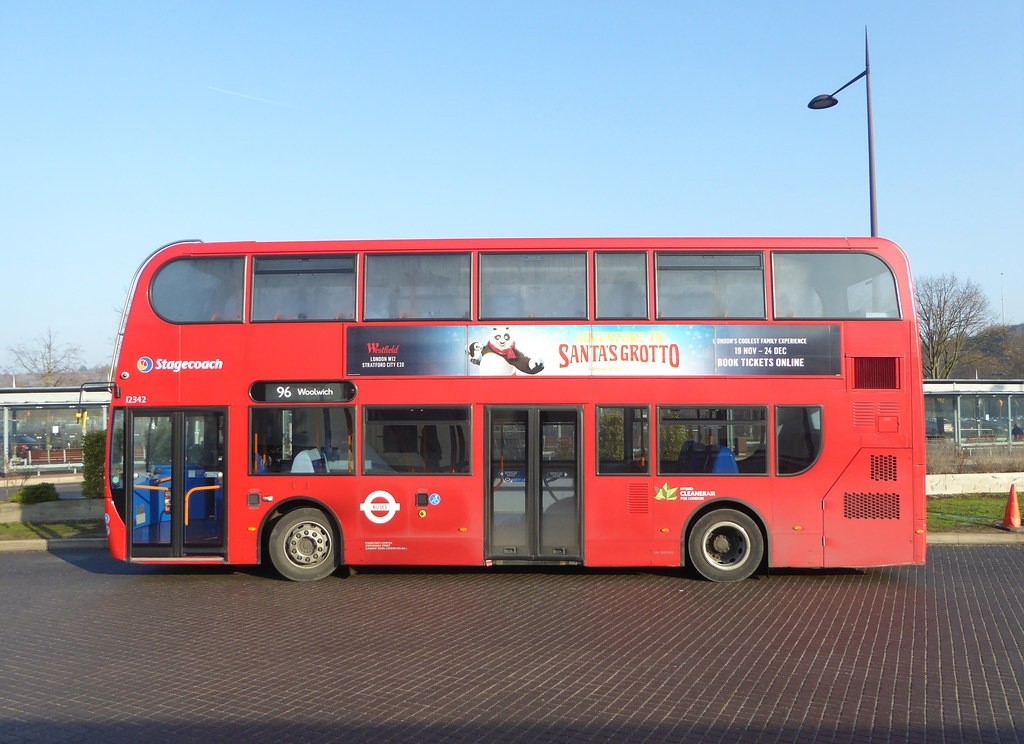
[806,27,882,310]
[993,483,1024,533]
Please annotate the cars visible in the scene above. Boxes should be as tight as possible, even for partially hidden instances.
[928,418,954,434]
[0,435,42,459]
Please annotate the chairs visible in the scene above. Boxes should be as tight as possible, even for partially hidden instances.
[289,449,330,475]
[211,311,407,322]
[676,440,739,473]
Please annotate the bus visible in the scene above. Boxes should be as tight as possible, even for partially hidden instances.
[75,236,927,583]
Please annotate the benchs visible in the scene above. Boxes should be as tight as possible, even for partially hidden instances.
[27,449,83,464]
[965,436,997,443]
